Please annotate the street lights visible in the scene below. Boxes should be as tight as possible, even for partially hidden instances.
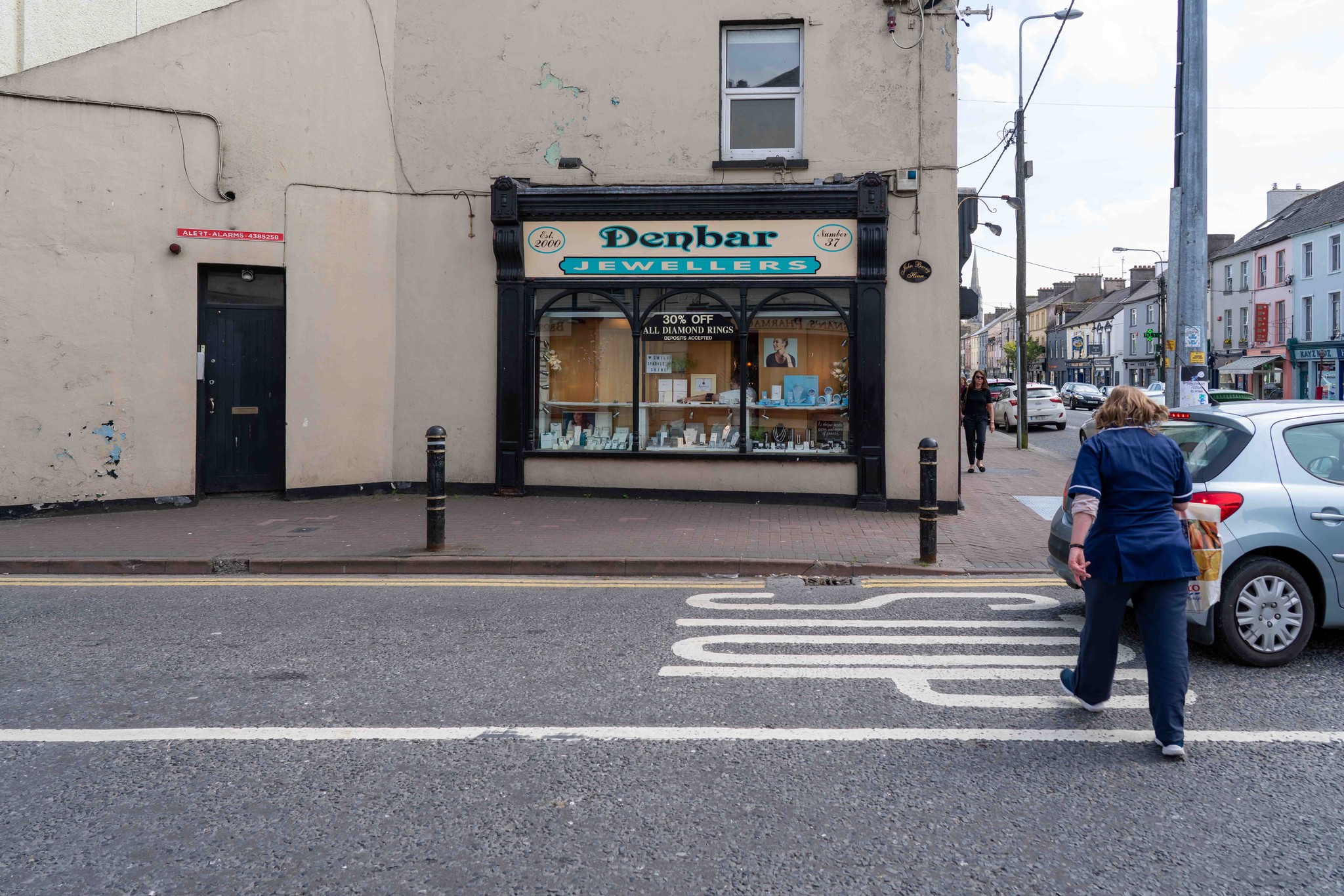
[1019,8,1089,448]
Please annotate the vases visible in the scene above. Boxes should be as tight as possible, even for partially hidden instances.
[549,370,559,402]
[838,386,846,404]
[591,366,602,403]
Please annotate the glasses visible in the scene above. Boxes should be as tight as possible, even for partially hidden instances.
[975,376,984,379]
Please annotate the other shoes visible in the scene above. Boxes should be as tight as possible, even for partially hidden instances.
[976,462,985,473]
[968,465,975,473]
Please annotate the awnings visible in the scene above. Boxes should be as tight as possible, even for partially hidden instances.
[1218,355,1284,374]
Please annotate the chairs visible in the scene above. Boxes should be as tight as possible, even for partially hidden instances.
[1179,441,1217,469]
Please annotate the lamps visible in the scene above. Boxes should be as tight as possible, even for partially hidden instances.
[1226,350,1241,354]
[1214,351,1226,357]
[688,408,694,419]
[1207,352,1214,358]
[977,195,1023,236]
[1092,324,1113,330]
[558,157,595,173]
[726,408,733,421]
[761,409,769,421]
[1330,334,1344,340]
[539,339,550,414]
[764,155,787,174]
[840,339,849,418]
[613,407,620,419]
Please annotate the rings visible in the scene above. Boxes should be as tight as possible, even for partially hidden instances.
[994,429,995,430]
[685,399,687,401]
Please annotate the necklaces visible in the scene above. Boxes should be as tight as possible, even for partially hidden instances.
[686,428,739,447]
[763,386,839,403]
[662,433,666,441]
[575,426,625,445]
[702,378,705,385]
[765,426,829,449]
[776,352,784,358]
[962,384,964,387]
[973,388,982,391]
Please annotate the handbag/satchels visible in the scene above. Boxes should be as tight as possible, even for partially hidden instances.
[960,400,966,426]
[1176,503,1224,615]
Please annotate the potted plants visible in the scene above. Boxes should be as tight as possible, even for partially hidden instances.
[666,353,698,374]
[749,422,767,442]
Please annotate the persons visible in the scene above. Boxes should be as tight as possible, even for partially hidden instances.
[960,370,995,473]
[679,374,758,426]
[766,338,796,367]
[1060,385,1201,755]
[568,413,594,435]
[960,376,968,427]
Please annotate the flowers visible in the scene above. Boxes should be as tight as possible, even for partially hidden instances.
[548,349,562,371]
[1223,338,1232,344]
[1238,338,1248,343]
[831,361,848,387]
[577,333,613,370]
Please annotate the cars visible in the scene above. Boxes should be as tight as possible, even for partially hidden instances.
[993,384,1066,433]
[1060,382,1108,410]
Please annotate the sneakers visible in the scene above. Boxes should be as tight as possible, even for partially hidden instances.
[1060,668,1106,713]
[1155,732,1189,756]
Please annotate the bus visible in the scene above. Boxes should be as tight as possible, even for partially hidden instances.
[1143,390,1166,406]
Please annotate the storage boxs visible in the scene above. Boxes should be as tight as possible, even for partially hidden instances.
[558,427,630,450]
[646,423,740,448]
[752,440,848,453]
[658,374,739,405]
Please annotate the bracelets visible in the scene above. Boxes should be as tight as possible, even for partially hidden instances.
[787,362,792,365]
[809,400,812,403]
[819,401,824,404]
[1069,544,1085,550]
[696,395,698,401]
[767,401,773,404]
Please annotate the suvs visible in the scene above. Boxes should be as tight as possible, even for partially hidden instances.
[1264,382,1283,397]
[1050,386,1060,397]
[1099,386,1115,398]
[1027,382,1041,384]
[1047,397,1344,668]
[1267,389,1283,400]
[987,378,1017,403]
[1148,380,1167,390]
[966,378,972,385]
[1208,388,1254,402]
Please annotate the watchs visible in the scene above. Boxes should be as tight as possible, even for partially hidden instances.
[990,421,994,423]
